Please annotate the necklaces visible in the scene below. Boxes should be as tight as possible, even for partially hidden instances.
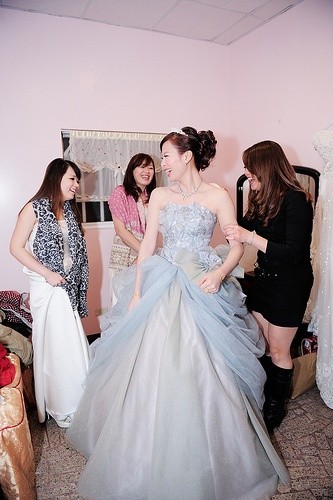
[175,181,204,200]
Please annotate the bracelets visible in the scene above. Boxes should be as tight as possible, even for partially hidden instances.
[132,293,140,296]
[248,230,256,245]
[212,270,223,281]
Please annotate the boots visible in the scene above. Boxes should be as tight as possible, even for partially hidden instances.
[261,358,297,437]
[256,354,272,374]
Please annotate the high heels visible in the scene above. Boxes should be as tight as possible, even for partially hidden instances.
[45,406,71,428]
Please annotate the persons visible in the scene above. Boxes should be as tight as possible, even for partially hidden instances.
[97,153,157,338]
[9,158,91,429]
[225,140,317,431]
[66,126,273,500]
